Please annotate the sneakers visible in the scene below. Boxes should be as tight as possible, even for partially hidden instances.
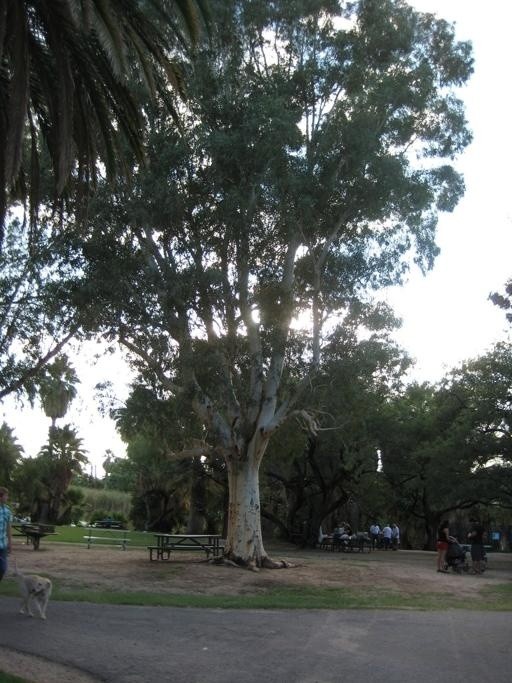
[436,569,449,574]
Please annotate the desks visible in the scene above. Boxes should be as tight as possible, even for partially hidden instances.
[82,527,130,550]
[154,534,221,558]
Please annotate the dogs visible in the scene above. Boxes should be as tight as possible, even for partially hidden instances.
[15,559,52,620]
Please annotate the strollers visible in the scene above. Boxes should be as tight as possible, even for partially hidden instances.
[443,536,469,573]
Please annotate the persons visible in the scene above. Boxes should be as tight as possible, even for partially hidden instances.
[436,518,487,575]
[318,521,399,553]
[0,487,13,582]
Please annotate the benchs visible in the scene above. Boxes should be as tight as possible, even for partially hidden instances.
[81,535,129,550]
[146,544,224,561]
